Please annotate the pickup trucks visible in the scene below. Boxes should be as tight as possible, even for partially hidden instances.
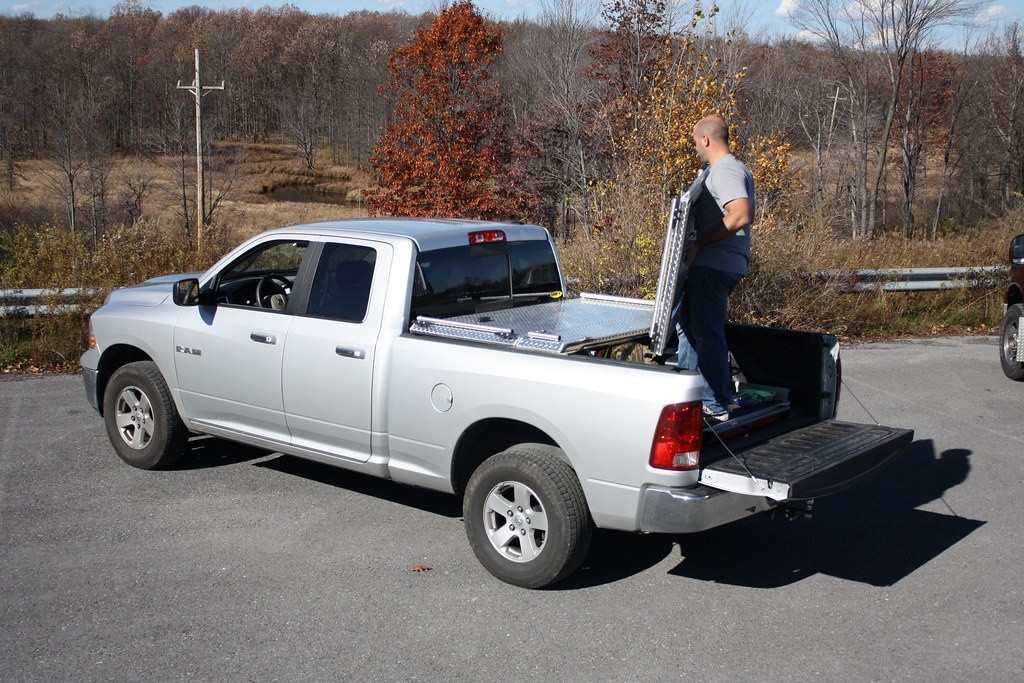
[80,217,914,589]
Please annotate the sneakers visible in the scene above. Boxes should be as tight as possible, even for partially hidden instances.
[701,405,730,426]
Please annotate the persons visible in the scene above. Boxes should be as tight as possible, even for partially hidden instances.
[678,115,754,421]
